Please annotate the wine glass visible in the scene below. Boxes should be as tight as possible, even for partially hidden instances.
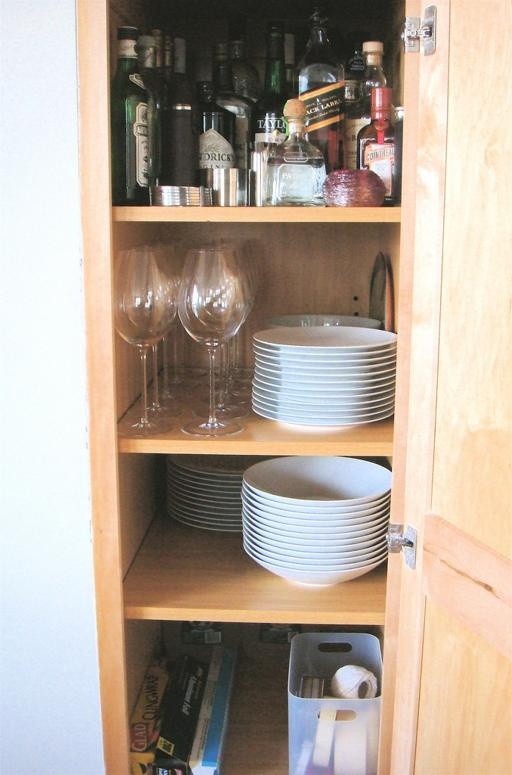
[112,236,257,438]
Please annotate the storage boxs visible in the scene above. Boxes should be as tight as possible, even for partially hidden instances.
[286,631,386,775]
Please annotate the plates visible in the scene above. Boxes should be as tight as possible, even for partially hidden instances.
[251,311,397,434]
[163,453,393,588]
[367,250,394,333]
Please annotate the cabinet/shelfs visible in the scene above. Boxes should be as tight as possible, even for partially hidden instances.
[78,0,421,775]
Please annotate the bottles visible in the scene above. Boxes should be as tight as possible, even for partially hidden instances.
[109,3,403,207]
